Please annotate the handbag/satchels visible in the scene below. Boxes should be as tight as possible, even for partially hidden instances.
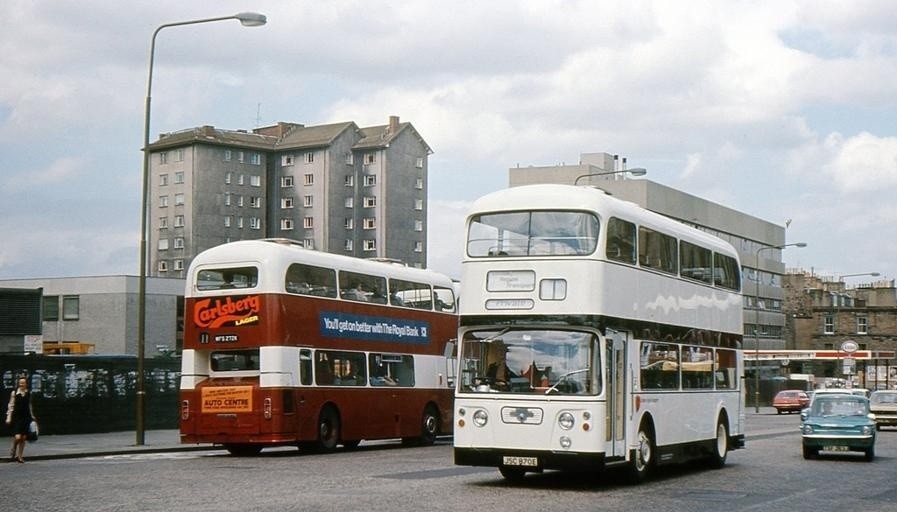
[24,420,41,443]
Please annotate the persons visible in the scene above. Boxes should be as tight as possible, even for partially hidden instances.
[5,378,38,463]
[821,403,834,417]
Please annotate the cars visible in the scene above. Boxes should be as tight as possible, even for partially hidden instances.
[800,389,877,461]
[773,387,808,412]
[868,389,897,429]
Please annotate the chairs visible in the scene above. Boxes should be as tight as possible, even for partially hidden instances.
[286,280,403,306]
[333,373,357,386]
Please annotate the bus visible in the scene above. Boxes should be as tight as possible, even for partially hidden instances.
[179,234,460,455]
[453,183,746,481]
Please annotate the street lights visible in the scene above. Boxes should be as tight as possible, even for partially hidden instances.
[135,13,267,445]
[836,271,881,388]
[573,167,649,183]
[755,242,807,412]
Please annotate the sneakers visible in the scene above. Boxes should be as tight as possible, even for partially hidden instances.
[9,447,24,463]
